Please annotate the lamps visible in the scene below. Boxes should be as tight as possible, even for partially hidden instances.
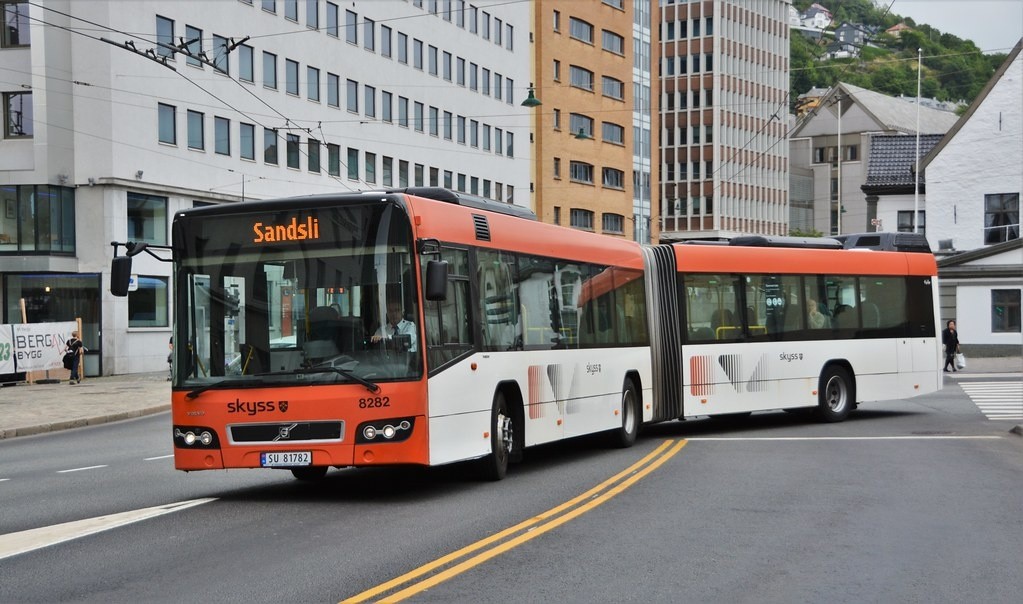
[520,89,543,106]
[574,127,588,139]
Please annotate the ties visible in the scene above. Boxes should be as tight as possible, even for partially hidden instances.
[393,326,399,335]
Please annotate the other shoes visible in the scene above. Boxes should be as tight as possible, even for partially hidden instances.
[943,368,950,372]
[69,378,81,385]
[953,368,956,372]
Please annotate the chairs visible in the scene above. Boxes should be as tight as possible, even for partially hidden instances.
[307,306,344,360]
[692,301,880,340]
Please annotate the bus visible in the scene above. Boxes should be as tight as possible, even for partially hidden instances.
[111,185,947,483]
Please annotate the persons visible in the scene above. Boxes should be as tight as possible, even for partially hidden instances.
[370,301,417,352]
[804,298,825,329]
[168,337,173,381]
[65,331,83,384]
[942,320,960,371]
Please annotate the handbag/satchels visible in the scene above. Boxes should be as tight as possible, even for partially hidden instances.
[956,353,966,369]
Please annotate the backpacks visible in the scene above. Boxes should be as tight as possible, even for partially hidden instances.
[67,339,79,361]
